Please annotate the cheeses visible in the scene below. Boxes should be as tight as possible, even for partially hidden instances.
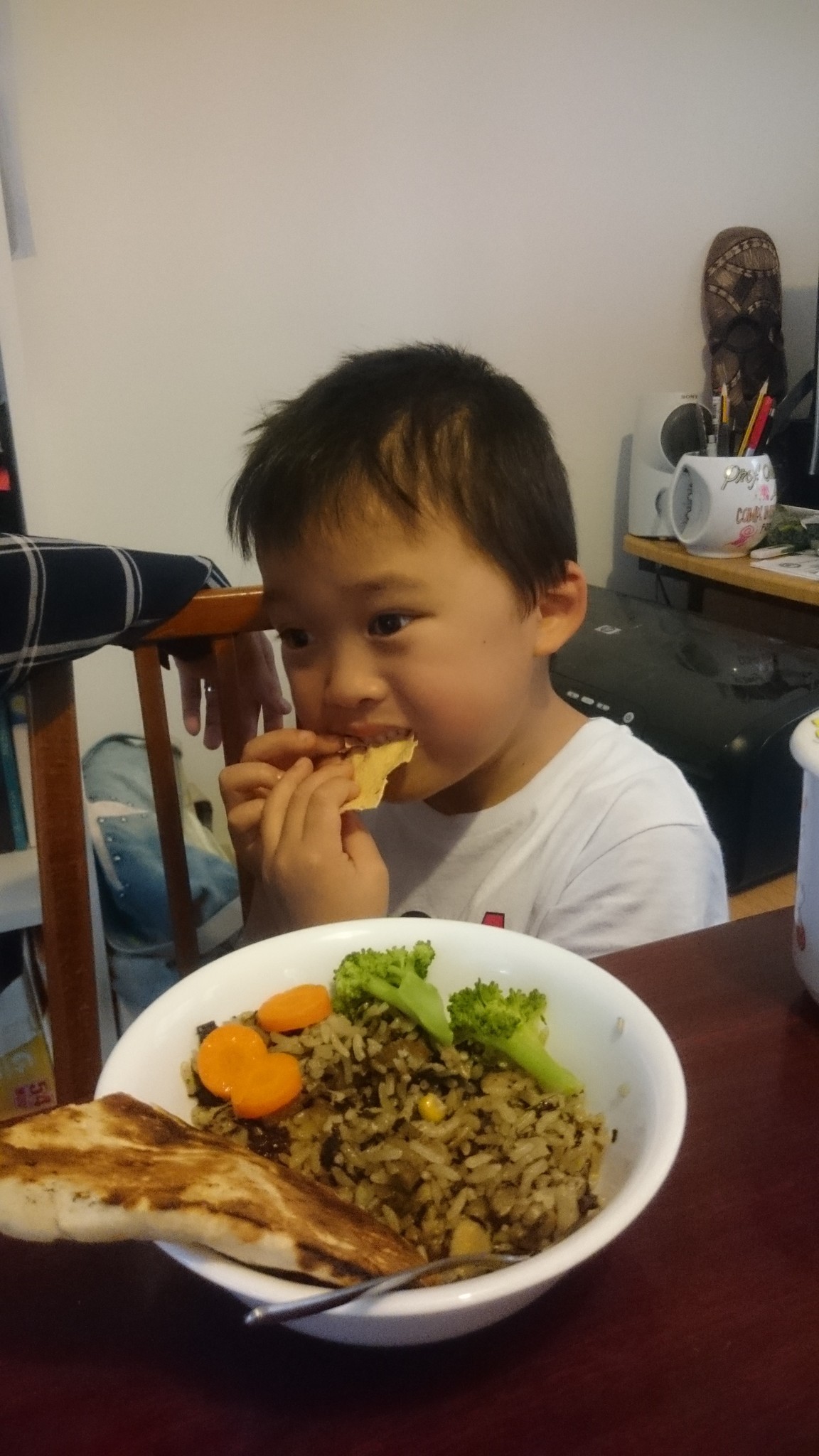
[339,735,417,816]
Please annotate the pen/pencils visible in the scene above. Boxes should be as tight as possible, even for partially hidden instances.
[694,375,777,457]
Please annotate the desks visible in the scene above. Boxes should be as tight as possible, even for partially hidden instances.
[616,529,819,618]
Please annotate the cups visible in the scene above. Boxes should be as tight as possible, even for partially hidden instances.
[788,711,819,1005]
[667,450,777,559]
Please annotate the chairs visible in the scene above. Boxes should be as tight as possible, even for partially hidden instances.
[26,584,297,1103]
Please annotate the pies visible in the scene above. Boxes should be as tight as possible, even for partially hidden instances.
[2,1094,433,1302]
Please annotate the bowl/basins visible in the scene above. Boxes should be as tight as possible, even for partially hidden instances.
[94,917,690,1349]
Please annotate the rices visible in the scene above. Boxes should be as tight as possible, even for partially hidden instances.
[195,992,602,1288]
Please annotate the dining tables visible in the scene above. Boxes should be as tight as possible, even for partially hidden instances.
[3,900,818,1456]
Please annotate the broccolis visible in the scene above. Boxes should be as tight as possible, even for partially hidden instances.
[331,938,591,1097]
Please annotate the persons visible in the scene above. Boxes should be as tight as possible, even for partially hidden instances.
[0,530,290,769]
[211,344,740,957]
